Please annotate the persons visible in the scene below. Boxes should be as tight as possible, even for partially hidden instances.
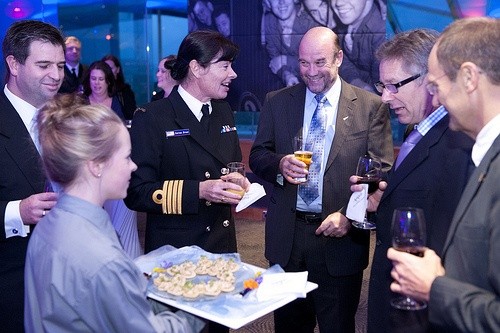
[387,15,500,332]
[248,27,389,332]
[58,36,90,94]
[123,30,251,332]
[101,55,137,119]
[0,20,66,333]
[82,61,125,124]
[24,99,208,333]
[350,28,475,333]
[189,0,385,113]
[151,54,179,103]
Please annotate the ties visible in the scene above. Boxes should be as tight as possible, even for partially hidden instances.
[394,130,423,172]
[298,95,328,205]
[72,68,78,81]
[200,104,211,132]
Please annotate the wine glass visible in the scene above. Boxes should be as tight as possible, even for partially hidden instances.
[349,153,382,229]
[389,207,429,310]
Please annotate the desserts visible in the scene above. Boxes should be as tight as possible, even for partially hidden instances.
[153,256,239,300]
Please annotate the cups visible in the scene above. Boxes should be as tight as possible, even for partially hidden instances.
[225,161,247,198]
[293,136,315,183]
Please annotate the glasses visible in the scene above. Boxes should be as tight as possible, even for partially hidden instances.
[373,71,427,93]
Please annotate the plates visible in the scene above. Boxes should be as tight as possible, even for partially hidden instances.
[145,253,257,304]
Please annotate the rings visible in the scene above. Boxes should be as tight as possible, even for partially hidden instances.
[221,196,224,202]
[42,209,46,217]
[331,234,335,236]
